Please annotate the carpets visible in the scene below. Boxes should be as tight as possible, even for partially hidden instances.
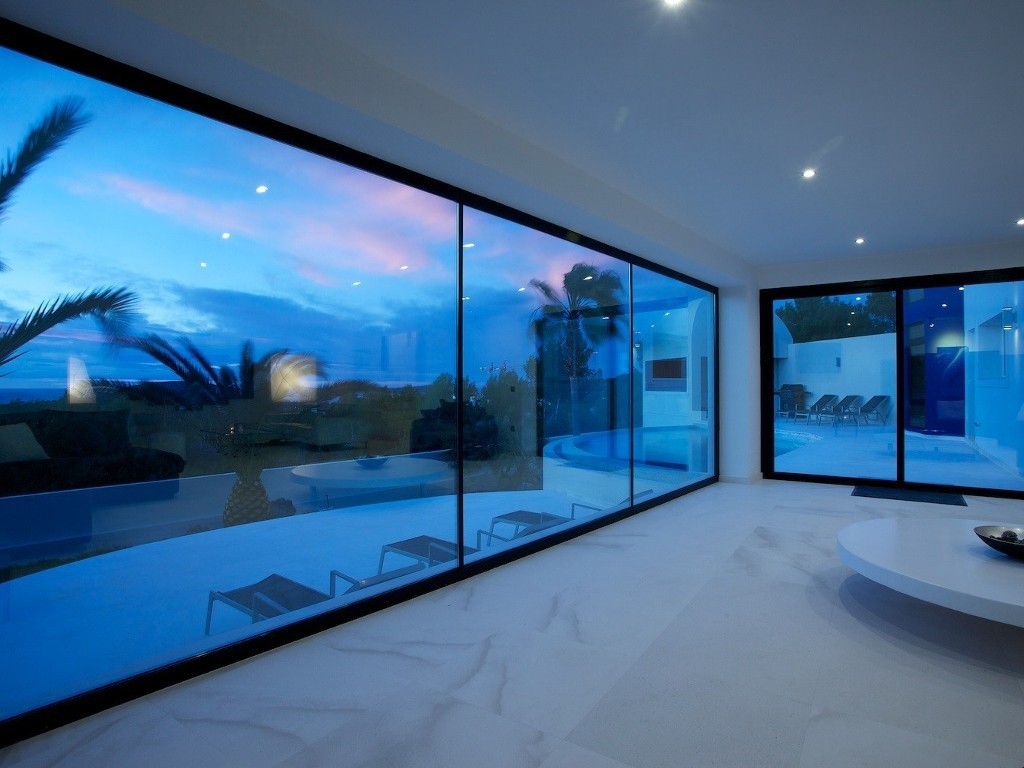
[851,485,970,507]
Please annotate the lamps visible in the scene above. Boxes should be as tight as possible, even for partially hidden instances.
[836,357,841,367]
[1001,307,1018,330]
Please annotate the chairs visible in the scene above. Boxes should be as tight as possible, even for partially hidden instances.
[775,394,890,428]
[206,486,655,635]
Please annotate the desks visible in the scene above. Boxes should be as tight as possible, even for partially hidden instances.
[837,513,1024,628]
[291,456,449,493]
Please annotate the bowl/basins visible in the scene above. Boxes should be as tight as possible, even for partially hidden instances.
[973,526,1024,561]
